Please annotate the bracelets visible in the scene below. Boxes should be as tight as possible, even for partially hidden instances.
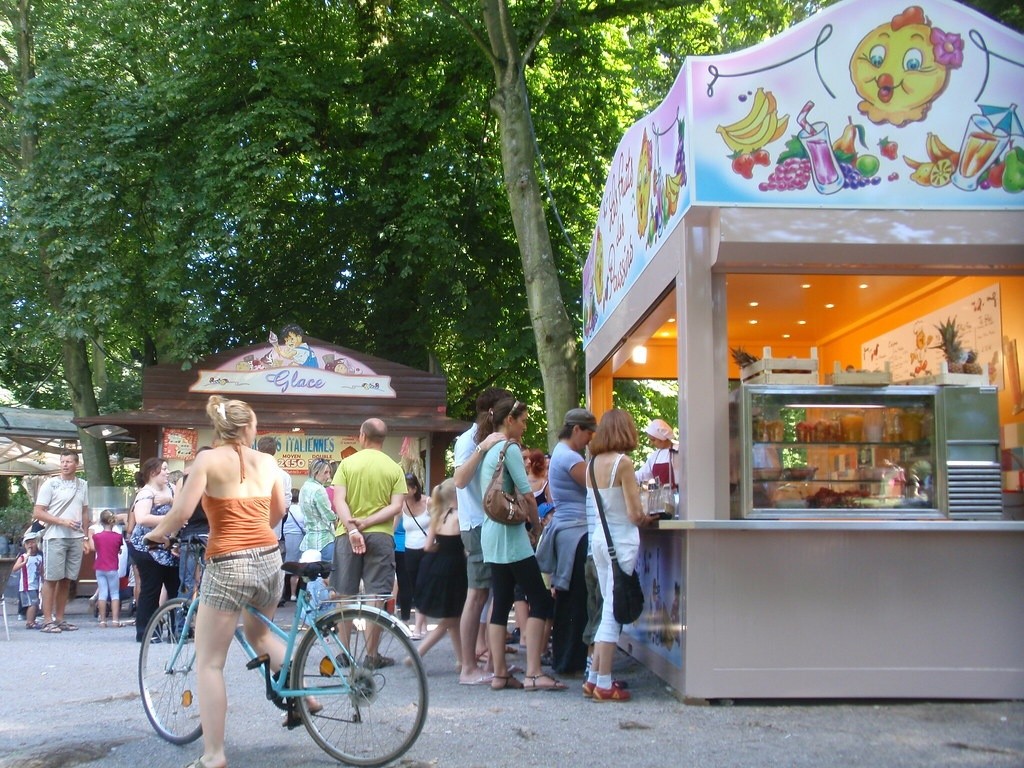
[475,444,484,454]
[348,528,359,536]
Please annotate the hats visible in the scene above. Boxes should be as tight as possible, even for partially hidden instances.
[539,502,554,519]
[564,409,600,431]
[23,532,39,544]
[645,419,673,443]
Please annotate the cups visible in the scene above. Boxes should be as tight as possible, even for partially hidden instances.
[753,407,922,442]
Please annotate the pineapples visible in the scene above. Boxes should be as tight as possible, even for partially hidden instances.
[931,318,983,374]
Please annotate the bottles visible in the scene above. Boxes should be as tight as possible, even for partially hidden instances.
[640,479,675,519]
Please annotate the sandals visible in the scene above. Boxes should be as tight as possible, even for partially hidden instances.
[523,672,565,691]
[491,674,523,690]
[18,607,125,633]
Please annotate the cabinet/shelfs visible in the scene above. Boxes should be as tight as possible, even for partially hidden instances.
[729,384,940,519]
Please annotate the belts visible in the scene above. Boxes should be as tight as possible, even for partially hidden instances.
[209,544,280,564]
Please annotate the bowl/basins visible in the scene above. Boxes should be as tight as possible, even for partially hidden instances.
[752,468,782,480]
[785,467,819,479]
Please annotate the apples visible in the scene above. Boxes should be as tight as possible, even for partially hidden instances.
[806,487,873,509]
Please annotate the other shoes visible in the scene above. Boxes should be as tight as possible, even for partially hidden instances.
[167,632,196,644]
[504,633,514,644]
[540,652,554,666]
[291,594,297,601]
[139,636,162,643]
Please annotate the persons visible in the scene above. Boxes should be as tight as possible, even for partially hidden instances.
[12,434,681,674]
[332,417,407,667]
[548,408,596,675]
[636,416,681,512]
[91,508,126,627]
[585,409,659,701]
[147,395,326,768]
[479,398,568,689]
[453,389,515,685]
[31,452,90,633]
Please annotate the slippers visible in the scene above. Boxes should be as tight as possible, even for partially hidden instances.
[459,674,492,686]
[412,630,421,640]
[505,645,518,652]
[475,650,489,663]
[281,704,324,728]
[184,759,204,768]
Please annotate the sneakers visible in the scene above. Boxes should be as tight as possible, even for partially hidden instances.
[336,656,350,669]
[582,675,627,697]
[364,653,394,668]
[593,682,630,702]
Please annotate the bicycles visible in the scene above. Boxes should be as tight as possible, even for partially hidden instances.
[137,535,429,768]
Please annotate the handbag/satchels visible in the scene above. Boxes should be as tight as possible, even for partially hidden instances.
[612,560,644,624]
[483,440,529,525]
[36,529,44,550]
